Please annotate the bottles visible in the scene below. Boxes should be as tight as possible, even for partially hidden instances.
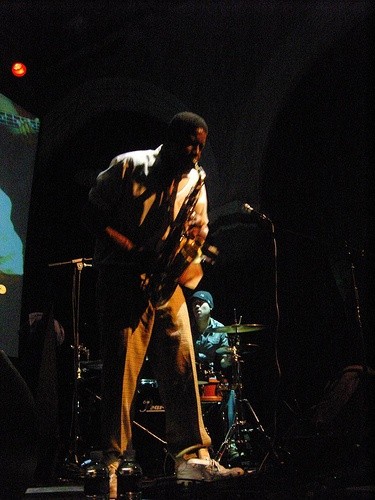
[116,449,142,500]
[81,451,110,500]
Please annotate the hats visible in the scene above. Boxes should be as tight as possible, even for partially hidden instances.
[189,290,214,309]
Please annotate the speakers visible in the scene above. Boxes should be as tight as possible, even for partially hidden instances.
[0,349,41,500]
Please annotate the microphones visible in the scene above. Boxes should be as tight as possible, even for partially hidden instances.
[240,203,272,225]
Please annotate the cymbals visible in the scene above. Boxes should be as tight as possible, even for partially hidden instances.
[215,343,263,353]
[209,323,267,334]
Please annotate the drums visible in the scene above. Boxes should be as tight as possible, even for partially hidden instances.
[135,378,167,412]
[198,381,223,403]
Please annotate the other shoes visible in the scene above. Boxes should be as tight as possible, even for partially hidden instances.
[176,459,245,485]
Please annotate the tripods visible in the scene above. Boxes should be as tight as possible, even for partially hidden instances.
[48,257,102,464]
[252,219,290,479]
[215,309,269,463]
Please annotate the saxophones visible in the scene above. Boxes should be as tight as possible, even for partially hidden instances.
[146,160,220,308]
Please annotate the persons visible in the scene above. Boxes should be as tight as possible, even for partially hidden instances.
[188,290,245,464]
[85,109,245,483]
